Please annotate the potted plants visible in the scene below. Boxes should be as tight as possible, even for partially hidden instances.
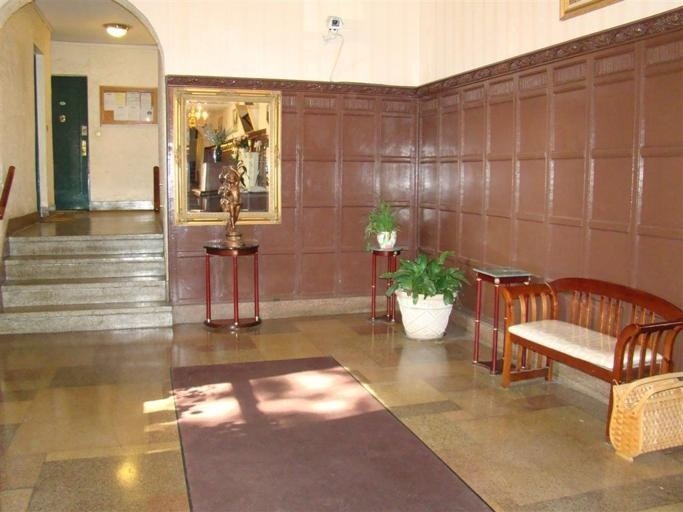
[367,200,401,250]
[380,246,470,344]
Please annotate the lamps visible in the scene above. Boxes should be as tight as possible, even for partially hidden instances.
[102,23,131,38]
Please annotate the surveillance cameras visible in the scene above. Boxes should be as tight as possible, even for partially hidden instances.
[330,16,343,33]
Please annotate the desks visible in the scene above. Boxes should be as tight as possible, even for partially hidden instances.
[199,188,269,213]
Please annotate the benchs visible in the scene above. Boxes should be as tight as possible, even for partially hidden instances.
[497,277,682,446]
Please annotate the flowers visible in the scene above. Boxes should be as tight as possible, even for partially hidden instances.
[234,135,249,148]
[207,125,233,146]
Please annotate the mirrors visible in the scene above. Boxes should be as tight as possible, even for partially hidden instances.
[173,85,282,227]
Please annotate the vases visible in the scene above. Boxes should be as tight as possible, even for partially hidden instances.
[239,146,249,153]
[213,144,222,163]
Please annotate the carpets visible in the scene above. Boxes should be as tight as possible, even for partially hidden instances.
[168,358,495,512]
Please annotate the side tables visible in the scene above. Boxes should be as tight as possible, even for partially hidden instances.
[202,238,261,330]
[369,250,402,324]
[466,266,533,374]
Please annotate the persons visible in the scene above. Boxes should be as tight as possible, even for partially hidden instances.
[216,174,226,212]
[224,165,242,234]
